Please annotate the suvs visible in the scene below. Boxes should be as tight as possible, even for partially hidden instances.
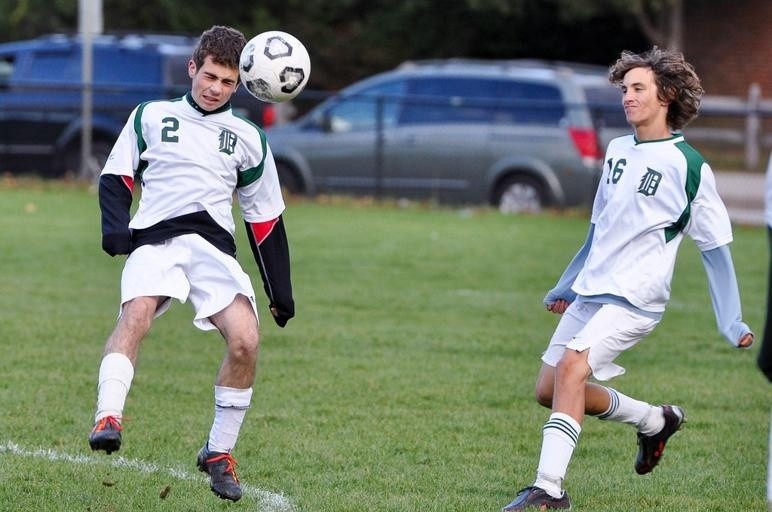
[0,30,293,179]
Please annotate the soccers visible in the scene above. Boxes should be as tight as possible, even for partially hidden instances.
[239,31,311,103]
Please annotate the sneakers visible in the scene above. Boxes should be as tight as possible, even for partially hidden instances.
[196,439,242,503]
[88,415,132,455]
[634,404,687,475]
[501,485,571,511]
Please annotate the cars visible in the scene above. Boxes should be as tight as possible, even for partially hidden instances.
[260,59,634,213]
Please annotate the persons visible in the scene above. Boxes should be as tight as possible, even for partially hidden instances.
[502,46,754,512]
[89,25,296,503]
[758,149,772,386]
